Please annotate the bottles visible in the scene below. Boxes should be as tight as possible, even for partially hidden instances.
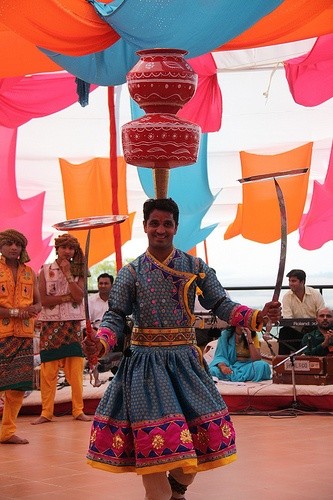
[121,113,201,167]
[126,48,198,113]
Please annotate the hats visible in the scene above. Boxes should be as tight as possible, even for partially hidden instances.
[55,234,91,277]
[0,230,30,263]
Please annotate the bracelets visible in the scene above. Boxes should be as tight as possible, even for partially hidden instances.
[67,274,75,283]
[249,340,254,344]
[9,308,19,317]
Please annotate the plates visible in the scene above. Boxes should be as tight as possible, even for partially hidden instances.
[52,215,129,231]
[237,168,309,184]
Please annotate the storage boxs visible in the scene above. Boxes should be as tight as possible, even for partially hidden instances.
[272,356,333,385]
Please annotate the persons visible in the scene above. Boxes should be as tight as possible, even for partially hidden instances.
[82,198,283,500]
[32,233,93,425]
[79,269,333,381]
[0,228,43,444]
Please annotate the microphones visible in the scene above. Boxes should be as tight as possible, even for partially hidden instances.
[243,329,247,337]
[308,337,312,351]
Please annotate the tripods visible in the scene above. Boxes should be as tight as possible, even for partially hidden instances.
[271,342,320,413]
